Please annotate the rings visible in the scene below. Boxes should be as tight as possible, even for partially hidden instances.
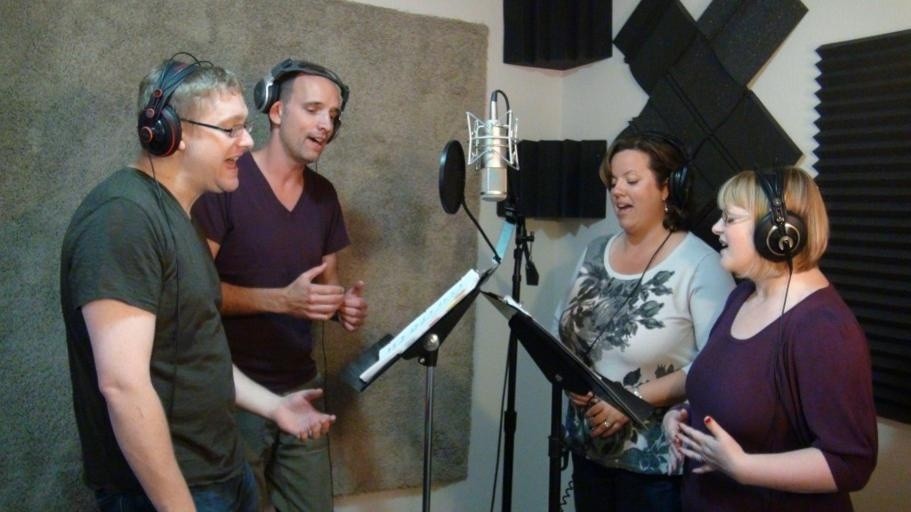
[604,421,612,429]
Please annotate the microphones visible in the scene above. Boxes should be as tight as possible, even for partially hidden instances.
[479,91,510,202]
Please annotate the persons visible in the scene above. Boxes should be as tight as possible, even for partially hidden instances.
[660,164,880,511]
[58,55,339,512]
[553,130,735,510]
[190,58,367,511]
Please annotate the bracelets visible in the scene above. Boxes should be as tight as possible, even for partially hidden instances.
[630,383,642,400]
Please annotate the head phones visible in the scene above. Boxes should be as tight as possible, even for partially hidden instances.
[253,58,350,145]
[642,133,696,206]
[137,51,214,158]
[754,165,807,263]
